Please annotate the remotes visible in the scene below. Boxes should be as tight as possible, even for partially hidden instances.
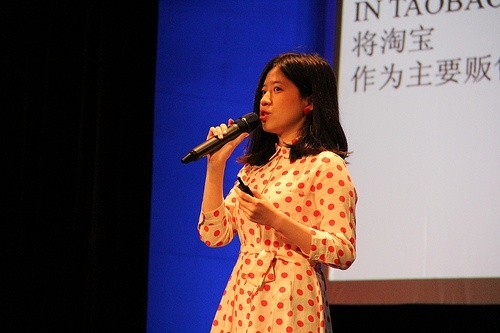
[236,177,254,198]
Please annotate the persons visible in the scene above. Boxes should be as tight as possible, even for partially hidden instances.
[198,52,357,333]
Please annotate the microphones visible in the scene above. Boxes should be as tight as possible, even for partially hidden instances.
[181,112,259,164]
